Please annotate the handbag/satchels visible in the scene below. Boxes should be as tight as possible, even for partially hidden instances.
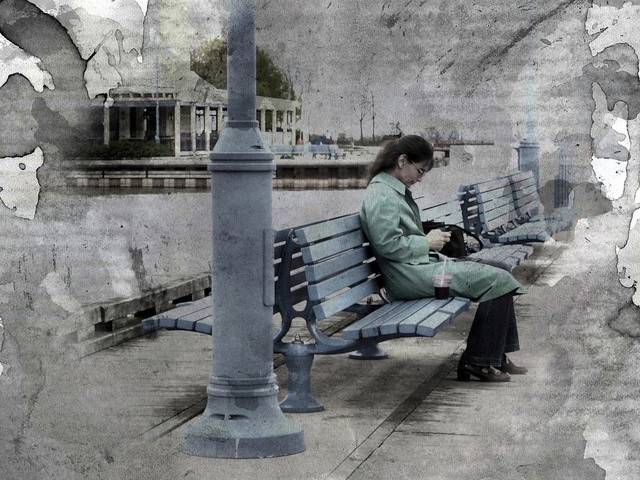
[420,221,483,258]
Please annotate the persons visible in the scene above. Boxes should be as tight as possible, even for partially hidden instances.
[360,135,528,382]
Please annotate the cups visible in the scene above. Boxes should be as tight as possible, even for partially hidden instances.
[431,275,452,301]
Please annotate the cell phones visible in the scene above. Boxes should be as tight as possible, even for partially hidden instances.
[440,226,459,232]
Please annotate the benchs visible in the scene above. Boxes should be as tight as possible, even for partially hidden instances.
[150,193,538,413]
[459,168,573,243]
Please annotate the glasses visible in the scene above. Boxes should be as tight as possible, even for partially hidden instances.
[408,158,426,177]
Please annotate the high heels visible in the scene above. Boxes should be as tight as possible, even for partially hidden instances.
[493,353,528,374]
[458,363,511,382]
